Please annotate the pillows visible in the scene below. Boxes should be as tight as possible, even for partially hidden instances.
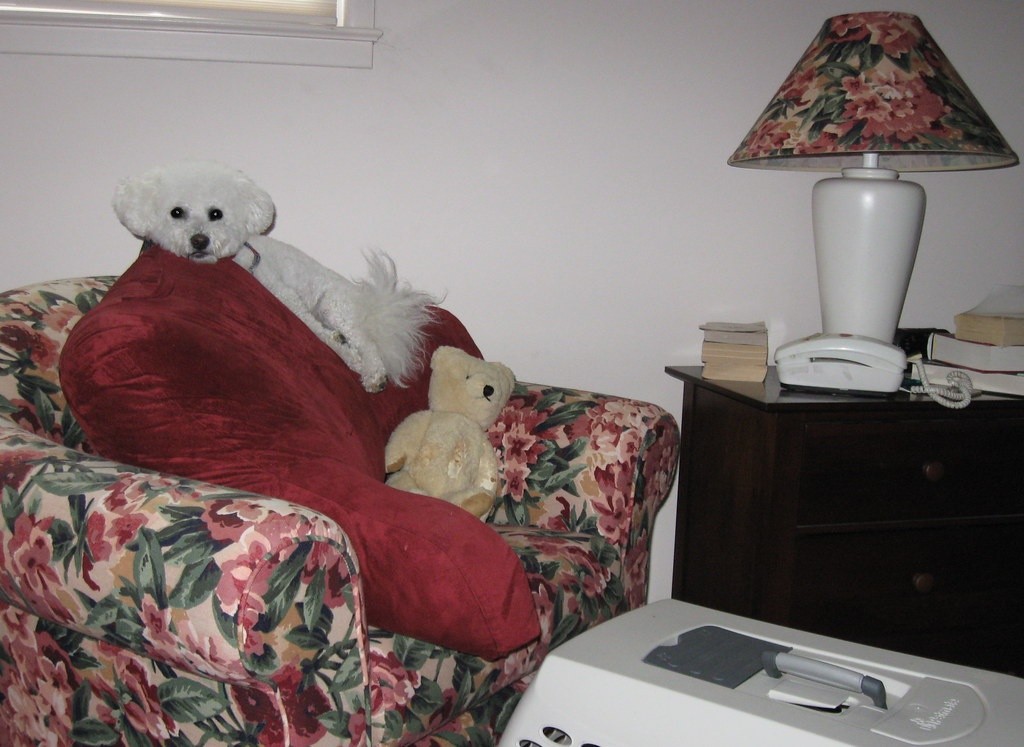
[57,242,539,662]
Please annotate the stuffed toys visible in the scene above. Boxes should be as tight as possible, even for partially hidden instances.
[382,343,518,526]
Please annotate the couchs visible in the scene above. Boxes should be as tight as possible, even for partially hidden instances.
[0,274,680,747]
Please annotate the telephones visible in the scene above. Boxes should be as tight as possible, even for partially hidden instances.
[773,333,907,397]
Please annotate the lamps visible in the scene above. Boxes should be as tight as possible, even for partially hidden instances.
[726,11,1021,346]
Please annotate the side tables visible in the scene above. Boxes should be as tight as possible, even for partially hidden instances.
[664,328,1024,682]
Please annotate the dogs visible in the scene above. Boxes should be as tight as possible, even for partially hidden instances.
[112,158,450,396]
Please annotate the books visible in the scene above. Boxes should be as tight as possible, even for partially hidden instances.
[696,320,771,388]
[894,285,1024,400]
